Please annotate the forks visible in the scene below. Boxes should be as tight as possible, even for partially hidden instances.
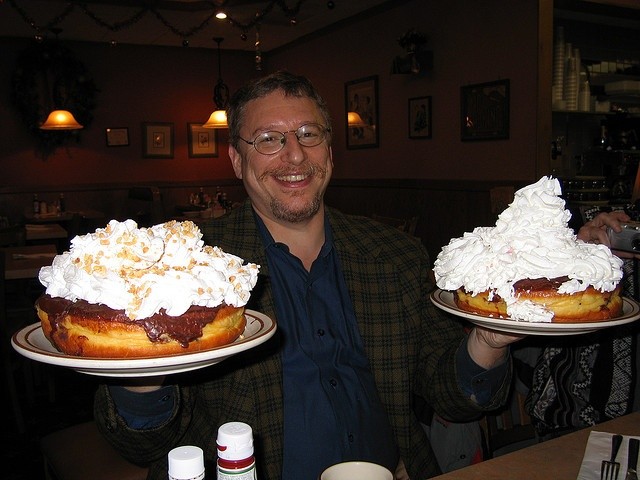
[601,435,623,480]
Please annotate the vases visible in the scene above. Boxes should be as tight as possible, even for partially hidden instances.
[408,52,421,73]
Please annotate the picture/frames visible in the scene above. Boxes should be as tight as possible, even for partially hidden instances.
[408,96,432,139]
[142,121,175,159]
[344,74,379,148]
[460,79,510,141]
[187,122,219,158]
[105,127,129,147]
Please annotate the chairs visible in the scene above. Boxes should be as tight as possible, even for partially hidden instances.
[480,389,542,461]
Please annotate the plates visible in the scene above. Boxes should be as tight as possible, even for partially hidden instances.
[12,309,277,393]
[430,288,640,336]
[182,212,199,217]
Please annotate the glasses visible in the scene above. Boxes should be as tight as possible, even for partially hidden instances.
[239,121,330,155]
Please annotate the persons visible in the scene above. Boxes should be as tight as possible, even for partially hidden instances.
[524,207,640,446]
[88,67,524,480]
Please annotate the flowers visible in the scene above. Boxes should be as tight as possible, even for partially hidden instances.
[396,29,429,51]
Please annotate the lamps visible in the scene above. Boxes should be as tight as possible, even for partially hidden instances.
[348,112,369,128]
[201,37,230,129]
[38,28,85,131]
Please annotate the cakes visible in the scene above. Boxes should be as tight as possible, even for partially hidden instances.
[435,176,623,321]
[38,220,261,356]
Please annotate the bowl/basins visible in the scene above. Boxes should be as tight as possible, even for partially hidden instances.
[200,211,211,218]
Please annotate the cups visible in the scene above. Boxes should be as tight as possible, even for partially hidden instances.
[319,461,393,480]
[552,24,591,113]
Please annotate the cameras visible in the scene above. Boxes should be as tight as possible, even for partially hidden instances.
[608,221,640,253]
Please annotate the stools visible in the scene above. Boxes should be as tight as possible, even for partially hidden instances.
[36,419,150,479]
